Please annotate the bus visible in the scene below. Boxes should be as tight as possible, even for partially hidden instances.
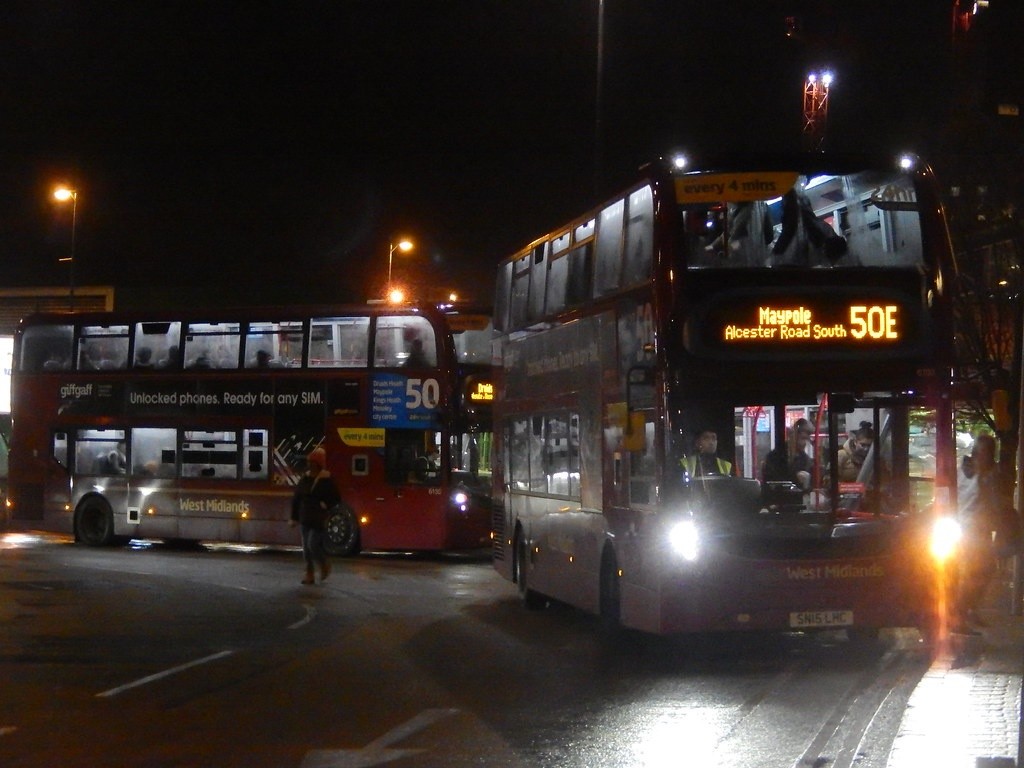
[490,117,956,666]
[8,311,492,553]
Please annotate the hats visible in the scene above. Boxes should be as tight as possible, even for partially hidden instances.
[307,448,326,468]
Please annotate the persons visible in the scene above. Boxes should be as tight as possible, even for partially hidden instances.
[399,339,431,370]
[42,336,273,374]
[679,409,898,524]
[414,445,459,479]
[289,448,343,585]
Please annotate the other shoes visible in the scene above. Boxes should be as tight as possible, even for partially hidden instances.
[320,559,332,580]
[302,569,315,584]
[968,619,990,629]
[951,625,982,636]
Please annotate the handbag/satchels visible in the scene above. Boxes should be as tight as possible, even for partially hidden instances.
[993,508,1023,560]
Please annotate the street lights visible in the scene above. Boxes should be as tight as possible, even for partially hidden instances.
[386,237,414,308]
[54,188,76,259]
